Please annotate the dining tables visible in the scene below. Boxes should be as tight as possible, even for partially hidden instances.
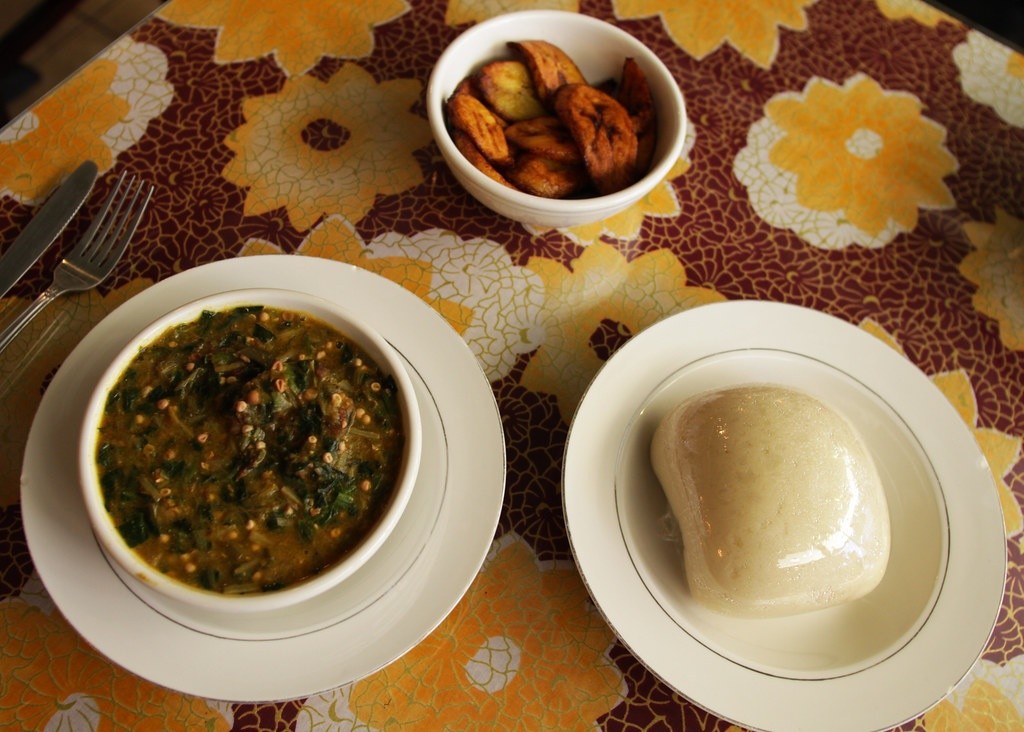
[0,0,1024,731]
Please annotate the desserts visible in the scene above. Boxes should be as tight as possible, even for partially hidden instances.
[650,386,891,622]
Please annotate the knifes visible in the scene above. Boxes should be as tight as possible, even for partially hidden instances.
[0,160,98,301]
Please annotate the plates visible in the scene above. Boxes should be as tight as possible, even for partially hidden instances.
[22,256,505,701]
[562,301,1007,731]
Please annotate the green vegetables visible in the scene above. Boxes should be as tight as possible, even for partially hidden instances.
[97,305,402,595]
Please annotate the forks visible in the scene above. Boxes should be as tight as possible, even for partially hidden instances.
[0,172,153,353]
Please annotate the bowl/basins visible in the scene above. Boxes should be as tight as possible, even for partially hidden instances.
[427,9,685,226]
[80,288,421,608]
